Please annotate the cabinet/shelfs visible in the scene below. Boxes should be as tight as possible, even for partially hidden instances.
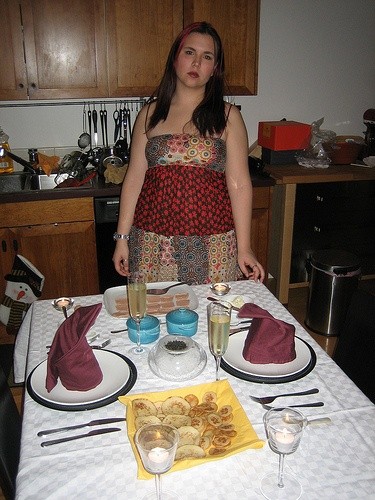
[0,193,100,344]
[106,0,260,98]
[0,0,107,100]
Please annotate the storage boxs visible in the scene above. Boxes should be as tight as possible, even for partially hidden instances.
[262,147,304,164]
[257,121,312,150]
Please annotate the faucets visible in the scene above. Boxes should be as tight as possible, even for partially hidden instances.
[0,148,43,174]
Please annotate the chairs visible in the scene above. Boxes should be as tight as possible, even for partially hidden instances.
[331,287,375,406]
[0,365,22,500]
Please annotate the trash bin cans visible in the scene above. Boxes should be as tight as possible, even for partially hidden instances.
[304,249,362,338]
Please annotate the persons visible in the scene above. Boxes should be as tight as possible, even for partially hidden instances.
[112,20,264,286]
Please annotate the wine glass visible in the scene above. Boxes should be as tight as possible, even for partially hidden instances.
[206,300,233,382]
[134,422,180,500]
[259,405,307,500]
[127,271,151,360]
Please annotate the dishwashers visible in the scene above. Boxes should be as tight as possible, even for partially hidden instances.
[95,196,127,294]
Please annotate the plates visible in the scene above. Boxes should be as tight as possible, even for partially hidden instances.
[218,295,255,313]
[148,342,208,382]
[102,281,198,319]
[215,327,317,384]
[27,347,137,412]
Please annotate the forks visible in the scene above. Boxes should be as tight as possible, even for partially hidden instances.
[262,402,324,412]
[46,339,111,349]
[87,334,100,344]
[249,388,319,404]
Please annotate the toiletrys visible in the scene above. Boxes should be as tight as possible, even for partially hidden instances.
[0,126,14,172]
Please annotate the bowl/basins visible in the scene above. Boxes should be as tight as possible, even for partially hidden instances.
[51,296,74,312]
[126,315,160,344]
[210,282,232,296]
[154,334,202,376]
[166,307,199,337]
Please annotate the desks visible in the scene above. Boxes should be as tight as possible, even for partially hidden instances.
[264,161,375,304]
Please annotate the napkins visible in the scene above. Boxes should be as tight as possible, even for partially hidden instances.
[45,303,103,393]
[238,303,296,364]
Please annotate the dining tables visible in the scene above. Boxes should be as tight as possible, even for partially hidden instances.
[13,280,375,500]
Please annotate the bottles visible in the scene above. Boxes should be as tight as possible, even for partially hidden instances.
[0,128,15,174]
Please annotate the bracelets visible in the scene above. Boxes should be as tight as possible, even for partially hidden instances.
[113,232,130,240]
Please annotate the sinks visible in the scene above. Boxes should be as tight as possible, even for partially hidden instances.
[0,174,27,194]
[27,170,93,192]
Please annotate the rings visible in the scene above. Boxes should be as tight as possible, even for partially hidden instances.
[251,264,257,268]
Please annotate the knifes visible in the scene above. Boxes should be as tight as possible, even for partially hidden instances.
[37,418,126,437]
[41,428,121,447]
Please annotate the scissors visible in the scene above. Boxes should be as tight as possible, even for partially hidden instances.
[113,111,125,143]
